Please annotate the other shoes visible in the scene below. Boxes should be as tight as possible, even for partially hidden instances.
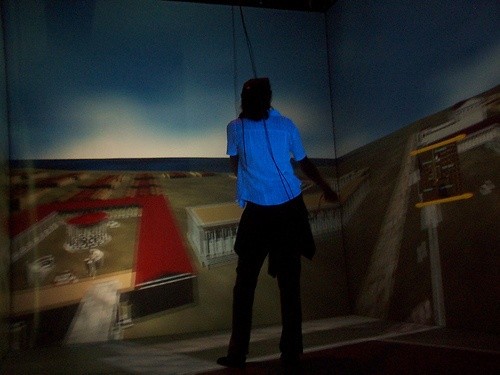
[280,351,303,364]
[216,357,247,369]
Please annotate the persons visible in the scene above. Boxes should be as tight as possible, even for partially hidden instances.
[215,77,318,375]
